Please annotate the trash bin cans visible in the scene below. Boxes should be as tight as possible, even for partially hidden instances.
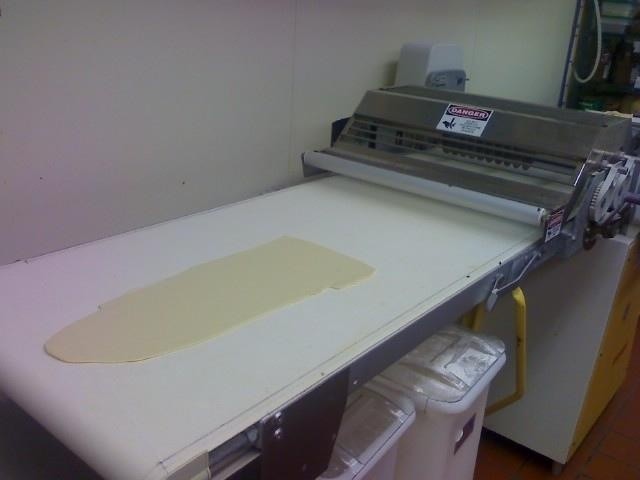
[371,321,507,480]
[311,380,417,480]
